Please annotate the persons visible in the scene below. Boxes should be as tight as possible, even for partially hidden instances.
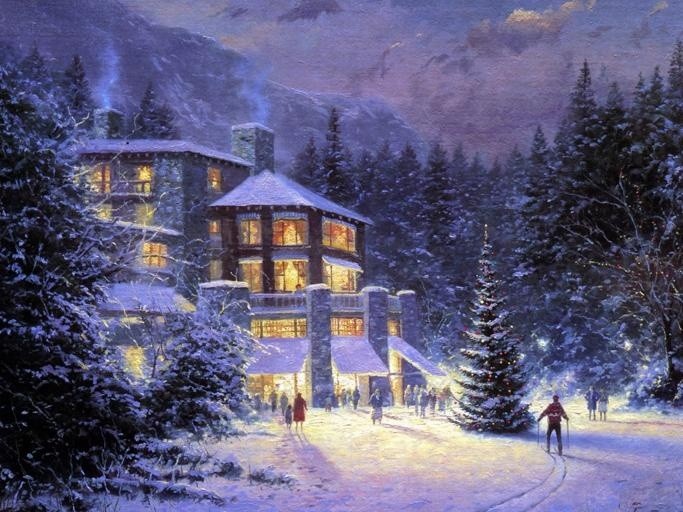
[321,388,361,412]
[404,384,452,418]
[584,386,600,420]
[367,389,383,423]
[537,395,569,455]
[293,393,308,429]
[268,390,277,412]
[598,389,609,421]
[281,392,289,416]
[285,405,293,429]
